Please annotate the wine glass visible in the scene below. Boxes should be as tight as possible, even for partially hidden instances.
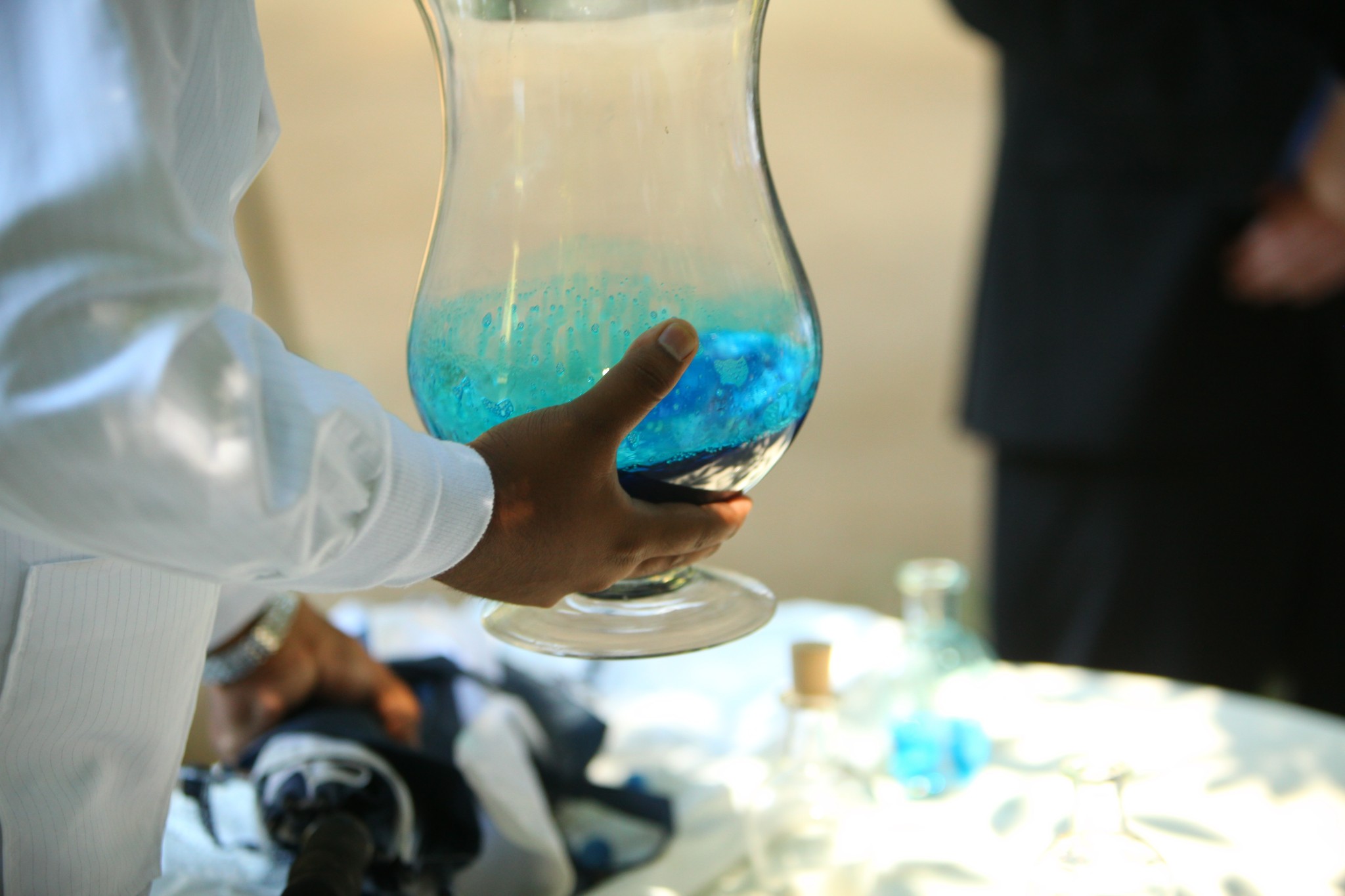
[407,0,822,660]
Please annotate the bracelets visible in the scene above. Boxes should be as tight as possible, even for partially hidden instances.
[202,591,300,687]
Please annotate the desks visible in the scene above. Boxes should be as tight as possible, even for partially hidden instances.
[153,593,1345,896]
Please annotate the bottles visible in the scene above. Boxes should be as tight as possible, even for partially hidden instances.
[888,555,993,794]
[765,634,863,890]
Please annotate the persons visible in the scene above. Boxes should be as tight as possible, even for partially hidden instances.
[0,0,753,896]
[945,0,1345,718]
